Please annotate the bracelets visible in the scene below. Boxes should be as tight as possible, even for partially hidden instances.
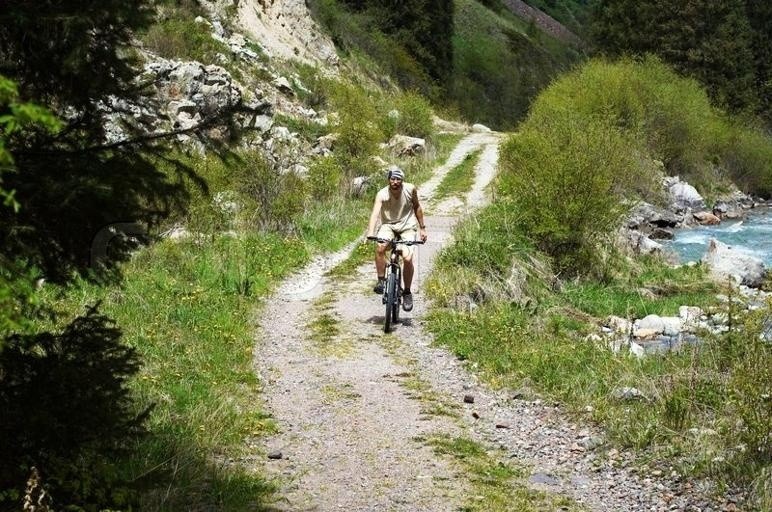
[419,225,425,229]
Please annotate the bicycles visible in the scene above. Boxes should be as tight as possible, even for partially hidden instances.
[370,236,423,333]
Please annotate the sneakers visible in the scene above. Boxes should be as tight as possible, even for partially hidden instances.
[374,281,384,294]
[403,292,413,312]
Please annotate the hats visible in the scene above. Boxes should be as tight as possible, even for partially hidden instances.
[388,168,404,179]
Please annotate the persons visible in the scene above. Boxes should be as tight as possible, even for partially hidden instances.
[362,168,425,311]
